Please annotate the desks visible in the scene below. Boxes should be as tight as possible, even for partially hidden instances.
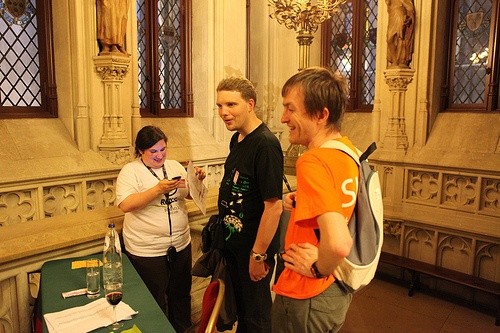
[34,252,177,333]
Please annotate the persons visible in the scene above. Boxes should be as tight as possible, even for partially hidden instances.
[116,125,206,333]
[217,75,284,333]
[385,0,415,66]
[96,0,129,54]
[270,65,369,333]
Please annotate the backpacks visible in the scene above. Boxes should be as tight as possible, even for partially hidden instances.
[313,138,384,295]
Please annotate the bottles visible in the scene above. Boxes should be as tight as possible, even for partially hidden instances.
[102,223,123,290]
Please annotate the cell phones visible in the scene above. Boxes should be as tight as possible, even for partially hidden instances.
[172,175,182,182]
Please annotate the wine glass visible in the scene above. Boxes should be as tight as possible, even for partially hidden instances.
[104,285,124,330]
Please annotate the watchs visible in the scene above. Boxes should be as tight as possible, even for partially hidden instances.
[250,250,267,262]
[311,262,330,281]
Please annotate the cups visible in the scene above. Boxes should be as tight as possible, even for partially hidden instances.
[86,257,100,299]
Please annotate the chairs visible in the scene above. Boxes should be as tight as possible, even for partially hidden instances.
[197,279,225,333]
